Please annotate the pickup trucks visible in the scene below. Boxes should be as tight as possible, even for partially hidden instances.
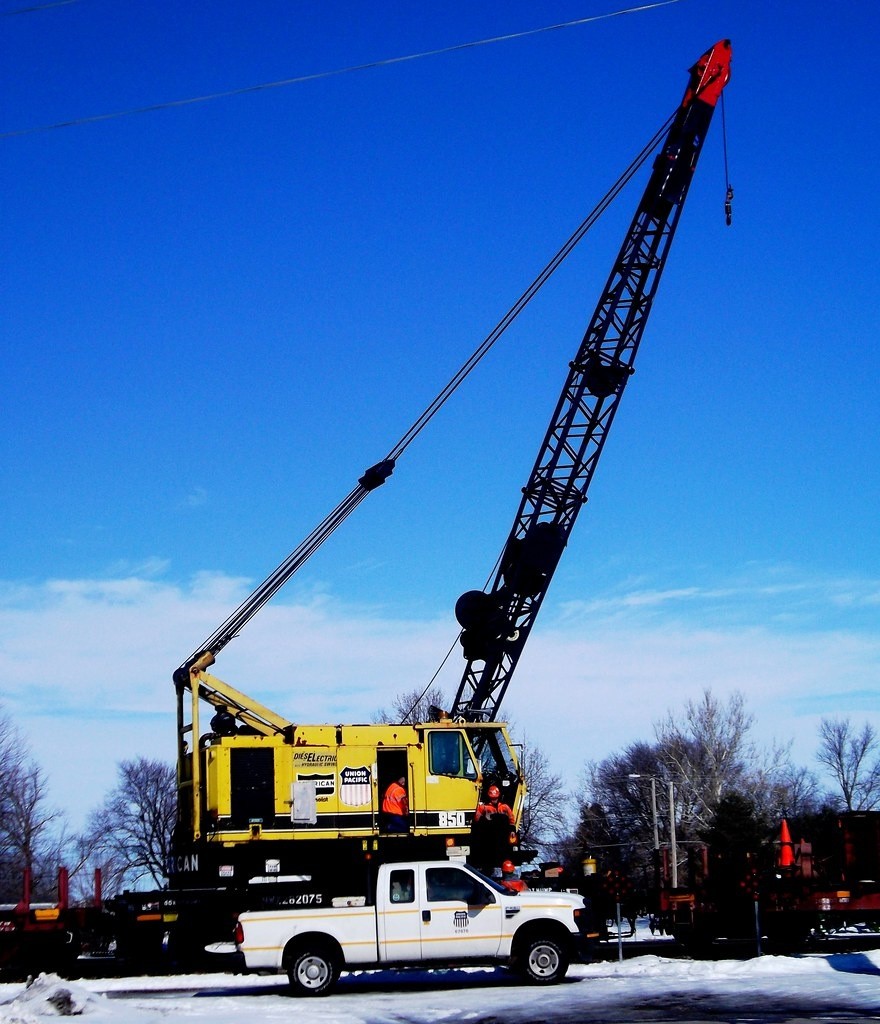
[205,858,612,1001]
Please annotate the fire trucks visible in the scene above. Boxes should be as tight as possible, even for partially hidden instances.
[647,772,880,960]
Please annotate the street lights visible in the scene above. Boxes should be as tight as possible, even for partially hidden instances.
[626,773,678,892]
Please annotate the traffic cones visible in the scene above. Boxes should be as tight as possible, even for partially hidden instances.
[777,818,798,868]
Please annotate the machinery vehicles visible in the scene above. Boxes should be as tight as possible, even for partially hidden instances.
[171,32,741,910]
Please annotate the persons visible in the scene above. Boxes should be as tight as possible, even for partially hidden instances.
[380,777,409,833]
[473,786,514,825]
